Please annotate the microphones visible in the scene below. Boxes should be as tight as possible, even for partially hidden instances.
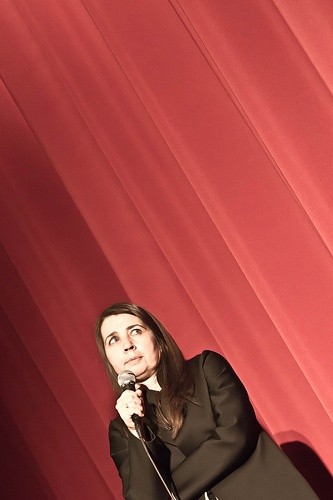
[117,370,144,438]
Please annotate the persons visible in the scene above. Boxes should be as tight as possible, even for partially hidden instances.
[95,302,321,500]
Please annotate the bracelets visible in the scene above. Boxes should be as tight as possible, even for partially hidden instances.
[128,426,136,430]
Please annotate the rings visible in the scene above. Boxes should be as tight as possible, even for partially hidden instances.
[125,404,128,408]
